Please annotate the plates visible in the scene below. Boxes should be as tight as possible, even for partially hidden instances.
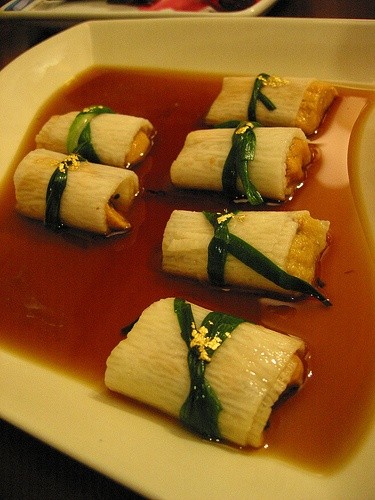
[0,19,375,500]
[0,0,278,31]
